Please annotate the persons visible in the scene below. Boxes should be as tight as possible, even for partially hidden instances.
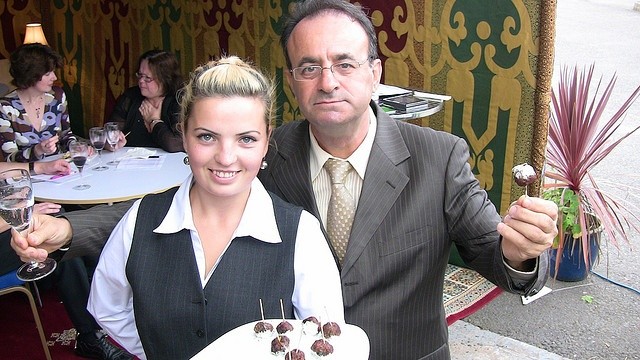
[85,55,347,359]
[0,160,134,359]
[98,49,190,153]
[256,0,560,360]
[0,41,96,163]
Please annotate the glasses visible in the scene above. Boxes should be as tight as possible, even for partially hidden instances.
[136,72,155,83]
[290,56,372,82]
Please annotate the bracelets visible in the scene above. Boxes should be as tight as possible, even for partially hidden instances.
[28,162,36,175]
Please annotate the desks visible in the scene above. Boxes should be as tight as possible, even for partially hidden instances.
[374,86,445,120]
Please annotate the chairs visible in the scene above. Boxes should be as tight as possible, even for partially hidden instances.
[0,269,50,360]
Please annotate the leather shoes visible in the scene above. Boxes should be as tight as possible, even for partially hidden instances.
[76,334,135,359]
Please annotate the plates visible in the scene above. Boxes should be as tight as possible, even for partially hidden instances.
[189,319,370,360]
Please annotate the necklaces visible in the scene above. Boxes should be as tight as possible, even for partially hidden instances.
[26,89,45,119]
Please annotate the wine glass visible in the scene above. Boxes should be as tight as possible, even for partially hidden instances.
[89,127,109,171]
[0,169,57,282]
[104,122,120,165]
[69,142,91,190]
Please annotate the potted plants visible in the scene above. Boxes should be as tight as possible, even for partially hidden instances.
[543,59,640,283]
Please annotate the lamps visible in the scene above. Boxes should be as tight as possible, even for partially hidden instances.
[23,23,51,49]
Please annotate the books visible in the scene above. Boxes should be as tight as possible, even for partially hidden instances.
[384,95,430,113]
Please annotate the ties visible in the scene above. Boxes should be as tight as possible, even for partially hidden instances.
[323,159,358,265]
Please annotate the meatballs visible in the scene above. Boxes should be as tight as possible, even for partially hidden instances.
[276,321,293,334]
[311,339,334,357]
[323,322,341,339]
[271,335,290,356]
[302,317,321,336]
[253,322,273,338]
[285,349,305,360]
[511,163,538,187]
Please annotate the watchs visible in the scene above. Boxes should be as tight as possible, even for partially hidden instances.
[148,118,162,133]
